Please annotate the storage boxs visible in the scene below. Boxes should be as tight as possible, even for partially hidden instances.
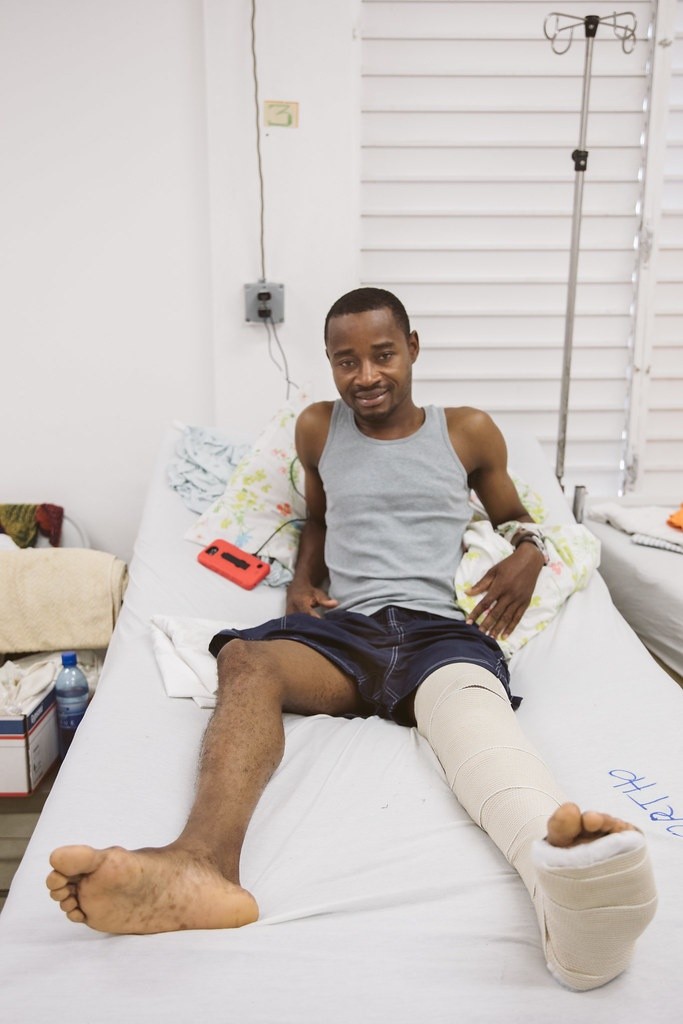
[0,692,58,800]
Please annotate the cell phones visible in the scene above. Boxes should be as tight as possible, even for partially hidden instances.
[198,539,270,590]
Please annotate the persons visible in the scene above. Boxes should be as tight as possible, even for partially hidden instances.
[46,288,657,992]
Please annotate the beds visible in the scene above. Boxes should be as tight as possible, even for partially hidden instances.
[0,438,683,1024]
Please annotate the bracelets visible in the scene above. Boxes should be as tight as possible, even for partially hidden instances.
[515,532,549,566]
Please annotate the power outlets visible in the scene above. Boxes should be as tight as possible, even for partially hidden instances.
[244,282,286,324]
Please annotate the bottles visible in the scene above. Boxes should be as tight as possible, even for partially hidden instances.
[55,652,89,762]
